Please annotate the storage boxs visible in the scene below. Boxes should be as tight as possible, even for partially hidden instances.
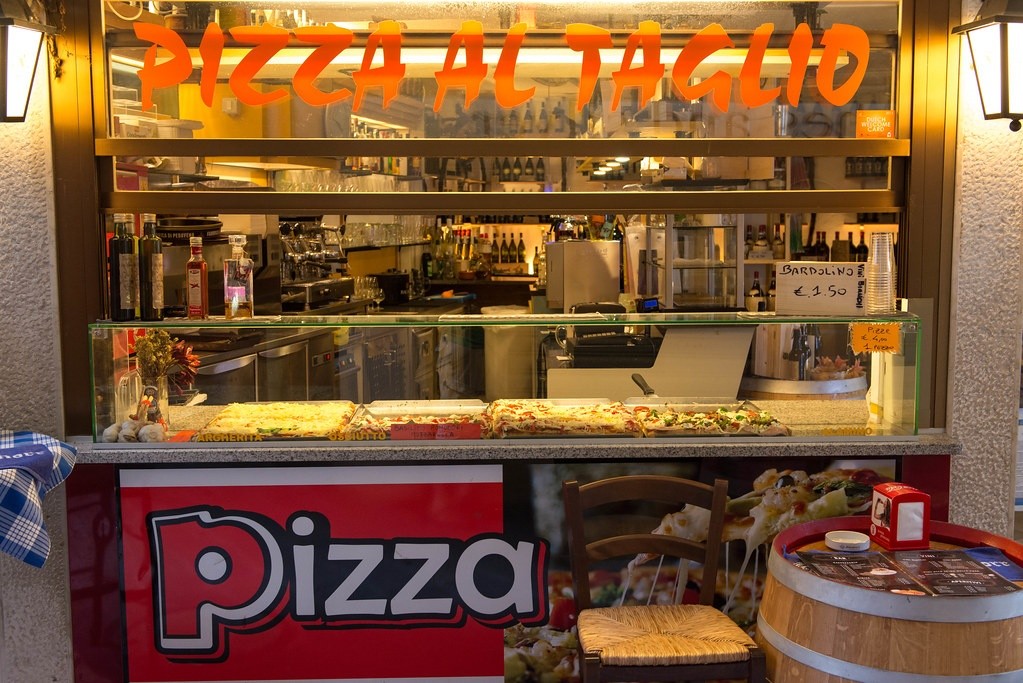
[868,481,932,551]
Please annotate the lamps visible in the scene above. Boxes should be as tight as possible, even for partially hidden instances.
[614,156,631,163]
[594,169,606,175]
[606,160,620,167]
[0,0,58,122]
[951,0,1023,133]
[599,165,613,171]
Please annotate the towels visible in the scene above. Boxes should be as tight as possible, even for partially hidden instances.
[0,428,78,568]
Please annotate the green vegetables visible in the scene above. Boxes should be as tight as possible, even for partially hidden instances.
[643,408,775,428]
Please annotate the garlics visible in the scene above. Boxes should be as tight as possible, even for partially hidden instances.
[102,420,166,443]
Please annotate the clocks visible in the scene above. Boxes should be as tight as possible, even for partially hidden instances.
[323,88,352,139]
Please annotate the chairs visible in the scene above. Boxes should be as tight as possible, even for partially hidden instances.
[561,473,766,683]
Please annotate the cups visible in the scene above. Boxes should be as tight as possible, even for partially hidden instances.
[864,231,898,315]
[343,222,423,246]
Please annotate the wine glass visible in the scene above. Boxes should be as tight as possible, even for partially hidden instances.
[354,276,385,311]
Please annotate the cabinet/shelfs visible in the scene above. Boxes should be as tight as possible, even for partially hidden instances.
[340,165,431,257]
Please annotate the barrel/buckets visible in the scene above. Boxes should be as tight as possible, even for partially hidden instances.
[738,372,867,399]
[753,516,1023,683]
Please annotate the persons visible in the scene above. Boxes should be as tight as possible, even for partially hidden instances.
[661,156,695,181]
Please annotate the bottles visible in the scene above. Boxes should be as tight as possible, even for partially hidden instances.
[746,271,766,312]
[185,237,209,320]
[766,270,776,312]
[138,213,165,322]
[109,213,135,322]
[422,213,553,286]
[223,234,254,319]
[742,221,898,262]
[125,213,140,318]
[342,99,564,181]
